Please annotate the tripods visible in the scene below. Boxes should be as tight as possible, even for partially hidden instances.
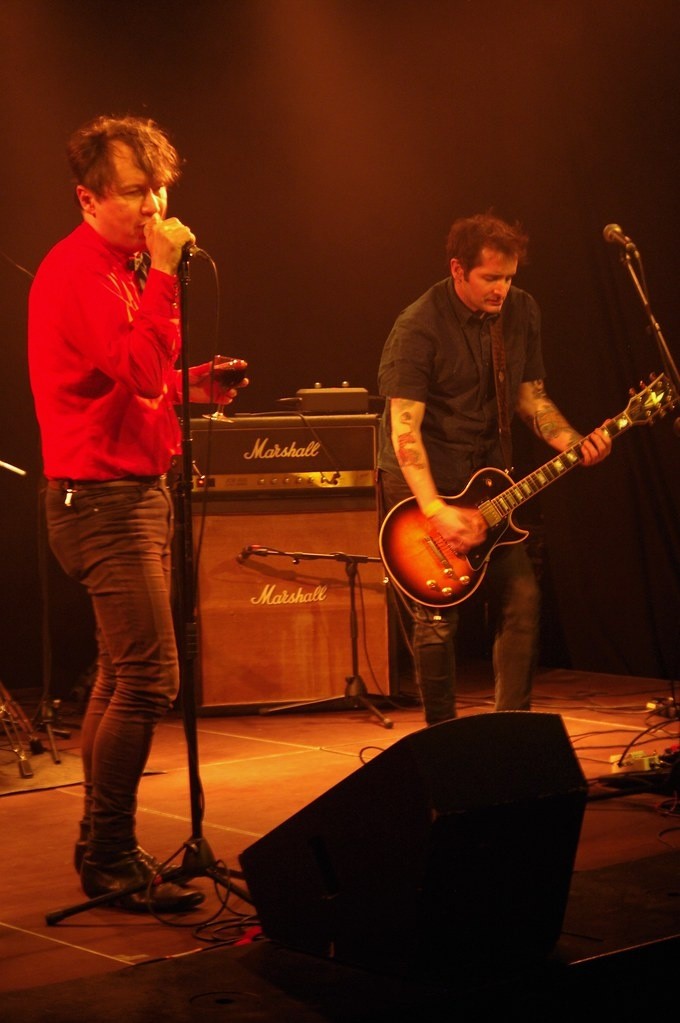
[43,252,261,929]
[23,475,83,766]
[252,547,399,729]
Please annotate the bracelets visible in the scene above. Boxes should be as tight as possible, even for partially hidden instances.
[421,499,444,520]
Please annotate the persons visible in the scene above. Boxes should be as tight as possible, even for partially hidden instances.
[375,210,613,726]
[28,124,252,912]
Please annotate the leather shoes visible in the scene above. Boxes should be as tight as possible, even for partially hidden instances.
[74,842,180,876]
[81,848,205,913]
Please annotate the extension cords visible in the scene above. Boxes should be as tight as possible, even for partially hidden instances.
[646,700,680,718]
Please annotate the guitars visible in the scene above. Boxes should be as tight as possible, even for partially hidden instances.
[376,370,680,612]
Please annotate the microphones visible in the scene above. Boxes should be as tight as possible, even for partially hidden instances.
[236,544,252,563]
[601,223,640,255]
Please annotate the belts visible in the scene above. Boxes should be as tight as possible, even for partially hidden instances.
[48,476,160,491]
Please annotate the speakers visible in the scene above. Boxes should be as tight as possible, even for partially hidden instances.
[186,496,400,719]
[240,709,588,974]
